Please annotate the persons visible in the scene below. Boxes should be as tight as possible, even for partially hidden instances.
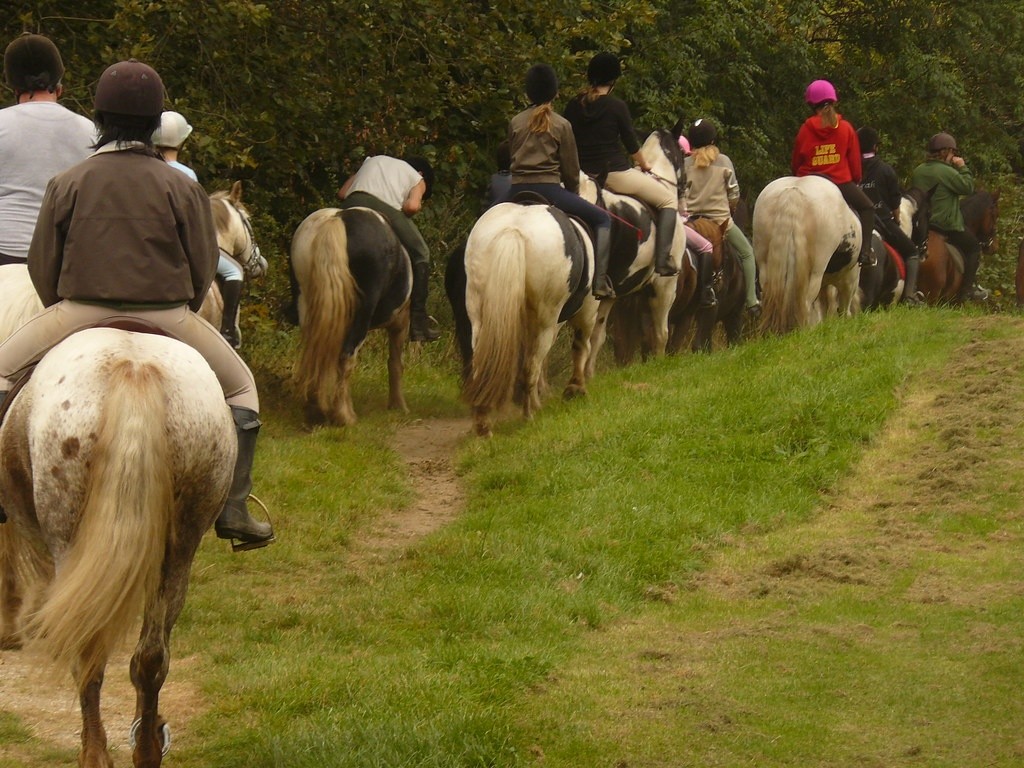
[854,127,988,304]
[792,80,874,266]
[286,155,439,341]
[483,52,761,315]
[0,34,272,543]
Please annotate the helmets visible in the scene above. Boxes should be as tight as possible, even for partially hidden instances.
[524,64,557,105]
[4,31,64,88]
[406,154,433,200]
[928,133,957,152]
[94,58,163,117]
[150,110,193,149]
[588,52,621,86]
[854,127,880,152]
[689,119,716,147]
[805,80,838,105]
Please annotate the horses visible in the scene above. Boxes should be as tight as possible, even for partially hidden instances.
[1015,237,1024,306]
[0,323,240,768]
[442,114,745,438]
[189,180,268,341]
[752,175,922,335]
[918,182,1002,307]
[288,203,411,430]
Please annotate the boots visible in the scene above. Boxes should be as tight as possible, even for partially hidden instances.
[592,228,616,297]
[218,279,241,346]
[697,253,717,305]
[286,274,299,327]
[959,252,983,305]
[653,207,677,275]
[900,256,925,305]
[215,405,273,545]
[410,263,441,342]
[857,206,875,265]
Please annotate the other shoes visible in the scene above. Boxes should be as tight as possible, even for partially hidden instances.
[748,301,763,314]
